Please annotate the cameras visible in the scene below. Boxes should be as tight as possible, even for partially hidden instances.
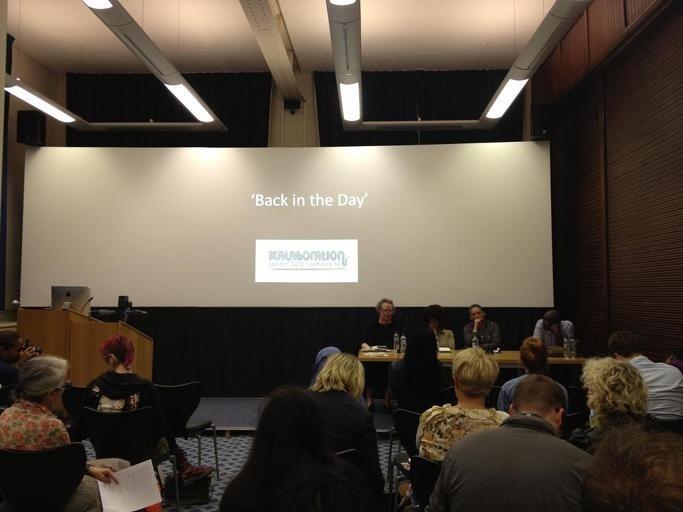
[24,338,42,356]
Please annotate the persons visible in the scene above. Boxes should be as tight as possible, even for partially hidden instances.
[0,330,37,408]
[217,299,683,512]
[85,333,214,487]
[0,356,133,512]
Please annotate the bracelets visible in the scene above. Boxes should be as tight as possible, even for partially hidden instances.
[85,463,94,476]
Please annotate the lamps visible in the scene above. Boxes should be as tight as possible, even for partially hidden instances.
[327,0,597,132]
[5,0,227,135]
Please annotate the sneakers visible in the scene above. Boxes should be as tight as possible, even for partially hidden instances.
[178,465,215,480]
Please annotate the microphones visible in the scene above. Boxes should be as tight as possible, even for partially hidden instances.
[80,296,93,314]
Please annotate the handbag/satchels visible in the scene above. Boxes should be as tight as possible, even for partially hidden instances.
[163,476,214,505]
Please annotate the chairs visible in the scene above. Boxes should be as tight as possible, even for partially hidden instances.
[334,386,682,512]
[1,378,220,512]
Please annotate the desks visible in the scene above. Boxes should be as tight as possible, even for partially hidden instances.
[359,349,587,376]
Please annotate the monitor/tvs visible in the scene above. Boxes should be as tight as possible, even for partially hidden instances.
[51,285,89,314]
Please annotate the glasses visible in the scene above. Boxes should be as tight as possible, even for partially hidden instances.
[63,380,72,390]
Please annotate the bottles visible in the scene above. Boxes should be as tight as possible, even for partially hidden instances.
[393,330,399,351]
[400,333,407,353]
[562,337,577,359]
[470,335,480,348]
